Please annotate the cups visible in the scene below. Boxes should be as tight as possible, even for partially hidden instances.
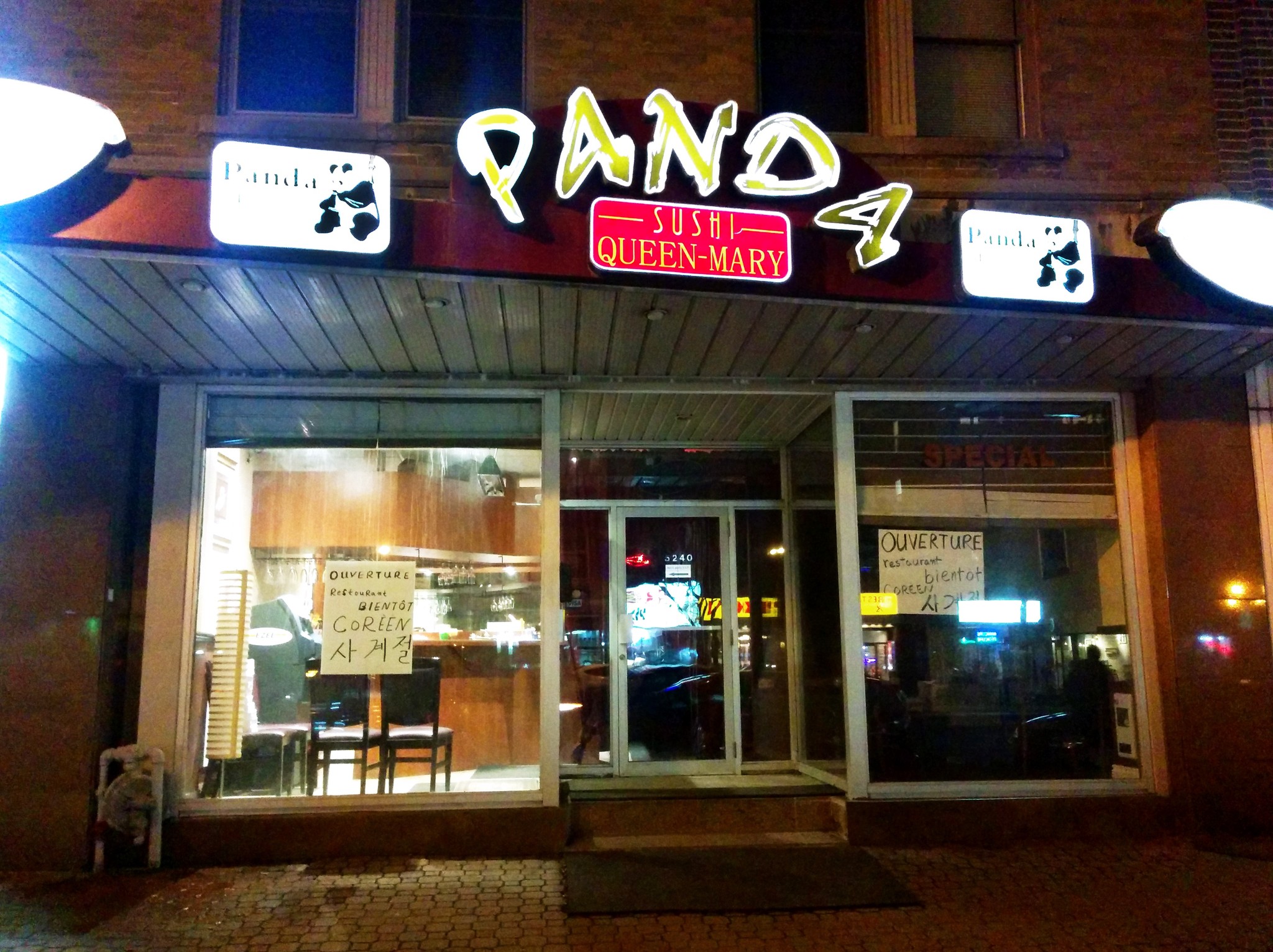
[439,624,451,639]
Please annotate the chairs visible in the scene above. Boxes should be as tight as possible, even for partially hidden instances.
[303,659,382,796]
[378,655,454,795]
[205,656,312,796]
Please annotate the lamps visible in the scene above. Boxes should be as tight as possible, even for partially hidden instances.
[477,448,507,498]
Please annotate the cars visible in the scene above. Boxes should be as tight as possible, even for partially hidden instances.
[565,662,1099,781]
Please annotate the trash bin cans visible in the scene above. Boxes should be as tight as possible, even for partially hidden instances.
[918,680,951,715]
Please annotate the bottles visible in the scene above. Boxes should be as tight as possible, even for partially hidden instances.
[575,630,600,647]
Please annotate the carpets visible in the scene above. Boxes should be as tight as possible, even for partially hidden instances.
[559,842,921,913]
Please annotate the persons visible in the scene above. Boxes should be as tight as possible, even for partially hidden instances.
[1064,646,1116,778]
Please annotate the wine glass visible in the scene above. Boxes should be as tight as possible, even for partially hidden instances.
[263,558,318,585]
[438,561,475,586]
[490,594,515,612]
[437,596,453,615]
